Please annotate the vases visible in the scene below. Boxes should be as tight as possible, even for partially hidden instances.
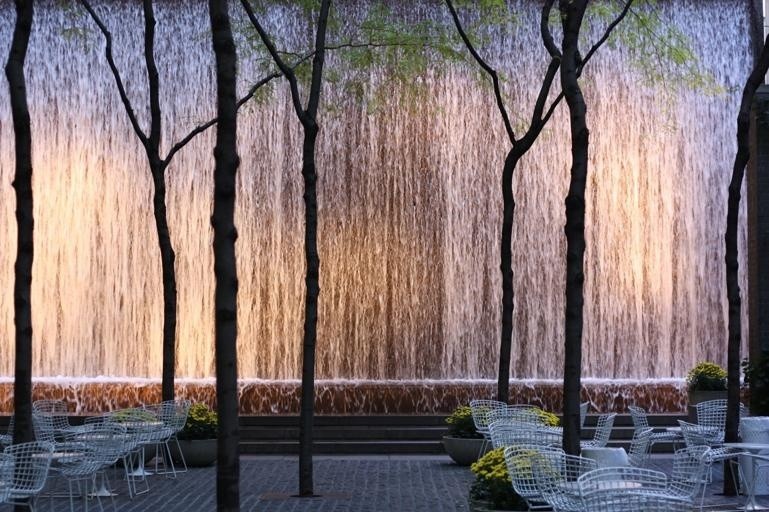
[688,389,728,415]
[440,435,493,466]
[164,436,218,470]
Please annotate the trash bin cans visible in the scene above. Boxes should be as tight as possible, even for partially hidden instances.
[741,417,769,496]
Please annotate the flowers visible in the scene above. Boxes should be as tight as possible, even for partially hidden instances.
[684,361,728,392]
[444,403,494,440]
[177,398,218,438]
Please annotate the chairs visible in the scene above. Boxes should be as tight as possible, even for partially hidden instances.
[468,398,769,512]
[0,394,192,512]
